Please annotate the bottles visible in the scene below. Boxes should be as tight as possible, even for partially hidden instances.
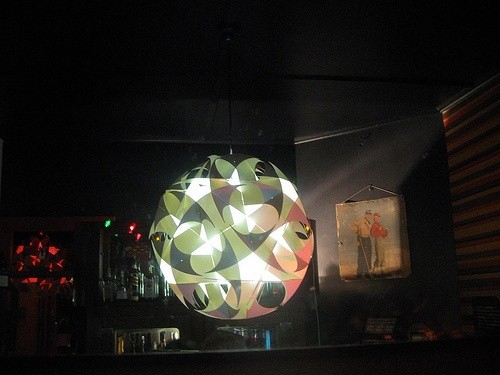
[109,249,170,300]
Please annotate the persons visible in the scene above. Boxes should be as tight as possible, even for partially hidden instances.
[370,213,388,277]
[353,210,374,278]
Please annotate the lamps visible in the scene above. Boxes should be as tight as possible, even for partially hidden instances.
[144,23,318,322]
[10,227,75,305]
